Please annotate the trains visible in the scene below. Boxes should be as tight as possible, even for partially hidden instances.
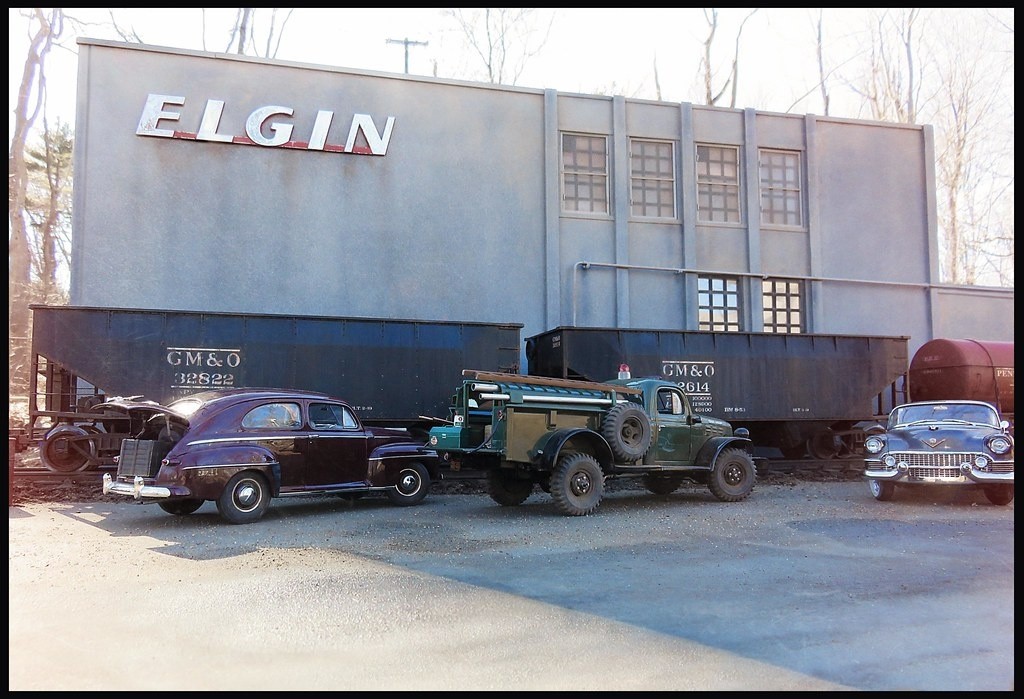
[10,302,1015,479]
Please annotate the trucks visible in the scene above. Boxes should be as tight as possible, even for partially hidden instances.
[427,362,770,517]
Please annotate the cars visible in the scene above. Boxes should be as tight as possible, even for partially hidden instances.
[857,398,1014,506]
[87,385,441,525]
